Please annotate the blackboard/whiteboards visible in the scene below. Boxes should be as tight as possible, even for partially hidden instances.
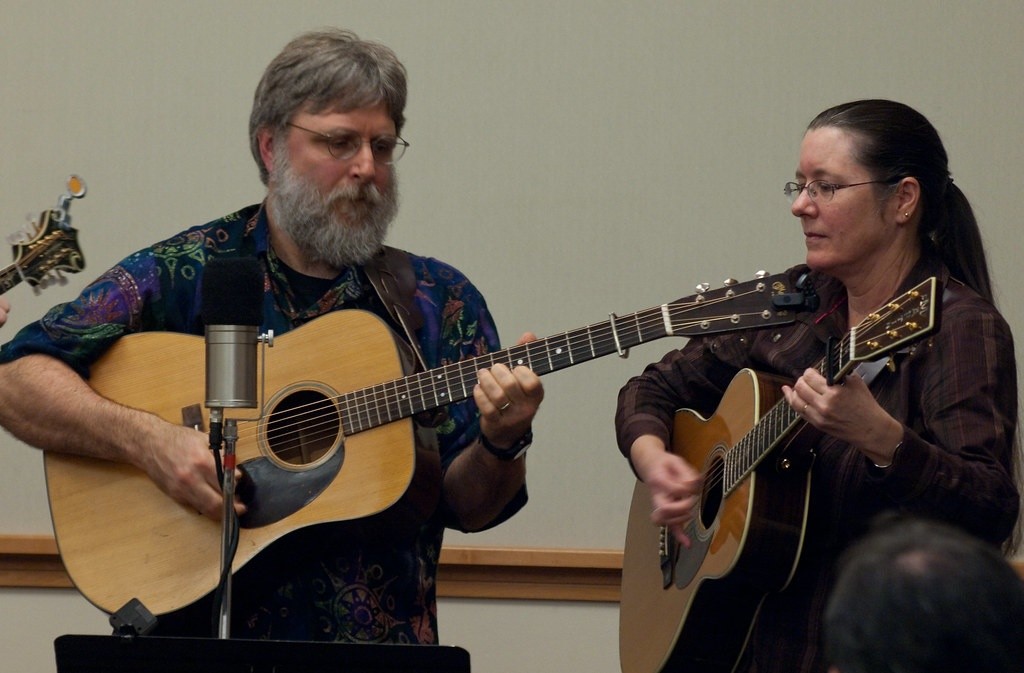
[0,0,1024,608]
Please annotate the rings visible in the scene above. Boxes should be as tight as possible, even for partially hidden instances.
[802,402,811,414]
[497,401,512,412]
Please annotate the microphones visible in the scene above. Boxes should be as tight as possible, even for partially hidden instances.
[199,257,263,407]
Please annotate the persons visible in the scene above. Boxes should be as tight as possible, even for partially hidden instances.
[1,27,552,650]
[611,101,1024,673]
[821,514,1023,673]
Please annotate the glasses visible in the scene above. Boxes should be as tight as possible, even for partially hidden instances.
[784,177,888,205]
[286,121,410,166]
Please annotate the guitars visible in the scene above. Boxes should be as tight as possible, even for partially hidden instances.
[44,261,822,622]
[0,173,87,297]
[619,274,946,672]
